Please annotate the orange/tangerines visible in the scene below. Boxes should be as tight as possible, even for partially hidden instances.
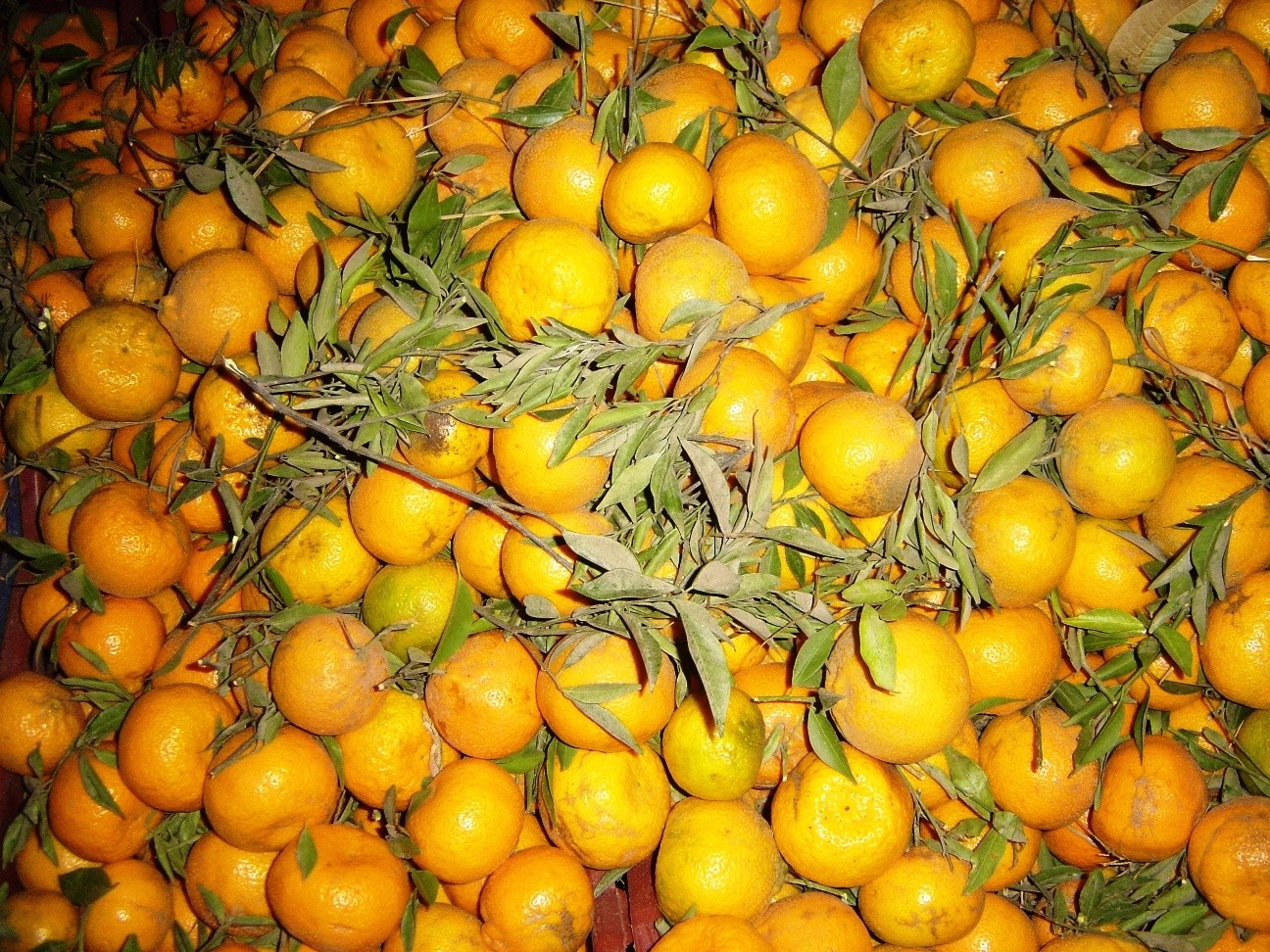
[0,0,1270,952]
